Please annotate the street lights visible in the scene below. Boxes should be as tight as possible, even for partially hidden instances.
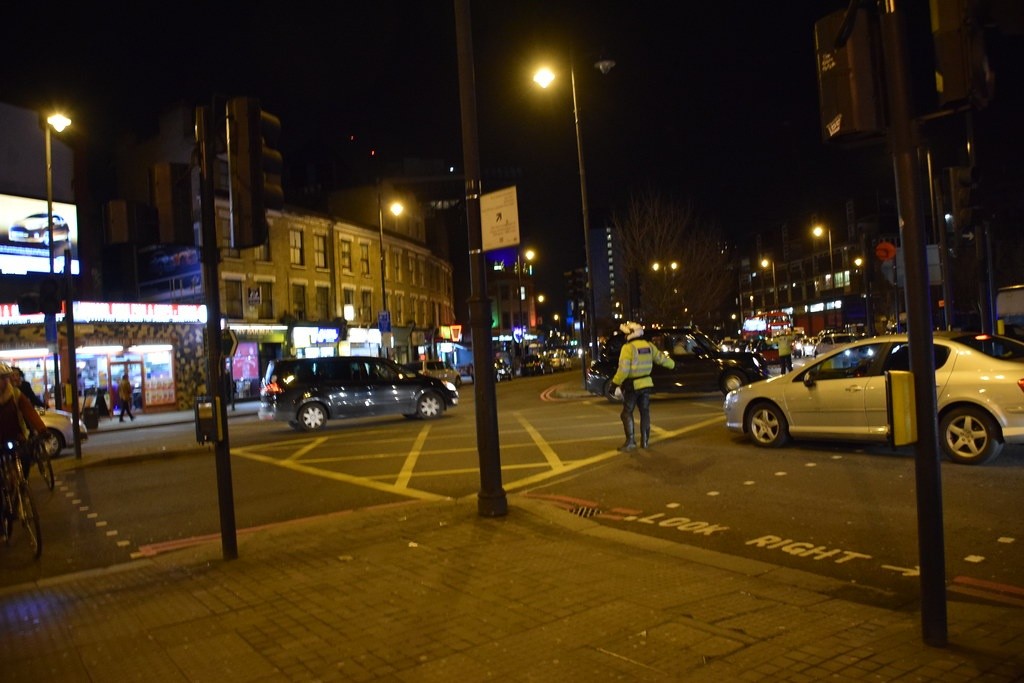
[814,224,838,326]
[518,248,535,328]
[651,261,680,310]
[761,257,779,307]
[44,111,71,410]
[377,194,403,311]
[532,56,598,366]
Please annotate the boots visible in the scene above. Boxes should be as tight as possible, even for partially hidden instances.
[617,416,637,451]
[641,415,650,448]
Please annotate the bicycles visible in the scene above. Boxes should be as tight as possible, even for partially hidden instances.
[0,440,43,559]
[31,435,55,491]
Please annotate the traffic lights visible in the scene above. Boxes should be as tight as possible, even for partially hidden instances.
[225,97,283,251]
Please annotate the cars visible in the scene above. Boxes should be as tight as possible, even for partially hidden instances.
[793,334,859,358]
[585,328,769,404]
[403,361,461,388]
[35,407,88,457]
[520,348,573,376]
[723,331,1024,465]
[469,359,512,382]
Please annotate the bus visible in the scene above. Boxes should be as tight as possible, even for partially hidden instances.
[740,312,791,339]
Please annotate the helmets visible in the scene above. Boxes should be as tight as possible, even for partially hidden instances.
[780,330,786,335]
[620,321,644,341]
[0,359,13,375]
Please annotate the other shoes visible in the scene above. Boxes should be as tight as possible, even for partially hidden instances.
[120,419,124,422]
[131,416,135,421]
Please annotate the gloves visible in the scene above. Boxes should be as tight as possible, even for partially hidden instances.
[608,382,619,396]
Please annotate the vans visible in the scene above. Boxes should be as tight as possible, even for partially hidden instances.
[257,356,459,432]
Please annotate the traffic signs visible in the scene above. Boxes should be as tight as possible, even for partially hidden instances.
[480,187,520,250]
[201,328,238,359]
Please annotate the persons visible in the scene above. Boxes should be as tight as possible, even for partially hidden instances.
[119,375,135,421]
[674,340,685,354]
[767,326,796,375]
[609,323,684,450]
[0,364,50,520]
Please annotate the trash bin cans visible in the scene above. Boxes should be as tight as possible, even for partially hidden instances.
[82,406,99,431]
